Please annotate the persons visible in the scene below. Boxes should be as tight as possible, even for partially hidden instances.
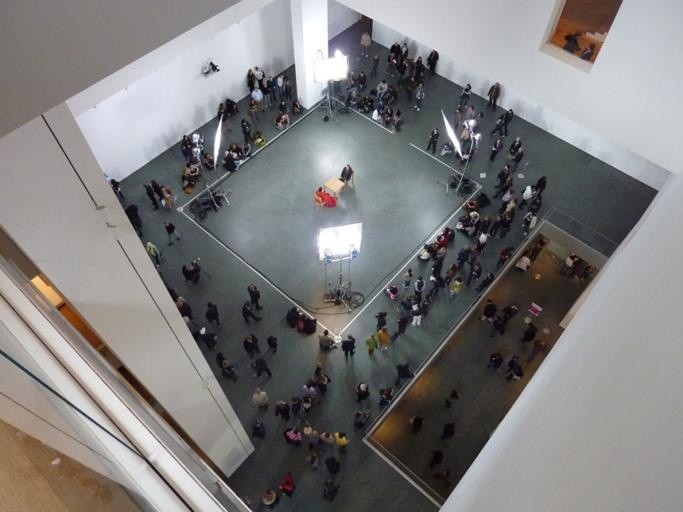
[110,30,596,511]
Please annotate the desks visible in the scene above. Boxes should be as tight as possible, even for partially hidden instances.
[324,177,345,199]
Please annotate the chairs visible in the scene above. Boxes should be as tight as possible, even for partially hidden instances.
[340,170,354,186]
[314,191,328,211]
